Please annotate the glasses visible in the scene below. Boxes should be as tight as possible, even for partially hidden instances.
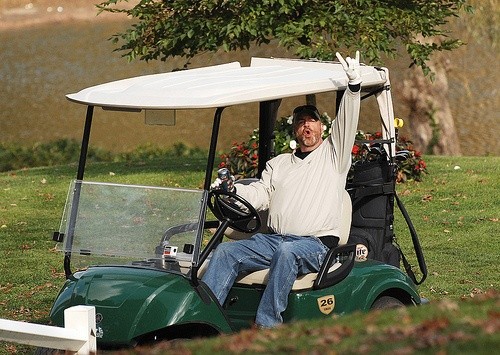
[293,106,320,115]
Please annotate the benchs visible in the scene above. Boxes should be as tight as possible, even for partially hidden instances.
[154,154,393,292]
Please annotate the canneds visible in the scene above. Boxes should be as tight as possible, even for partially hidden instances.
[216,168,231,190]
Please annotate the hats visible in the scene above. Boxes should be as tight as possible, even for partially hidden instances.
[293,105,320,124]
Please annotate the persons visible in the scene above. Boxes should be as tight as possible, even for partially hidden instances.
[201,50,364,329]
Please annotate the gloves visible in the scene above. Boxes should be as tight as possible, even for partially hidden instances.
[210,175,235,198]
[336,50,363,85]
